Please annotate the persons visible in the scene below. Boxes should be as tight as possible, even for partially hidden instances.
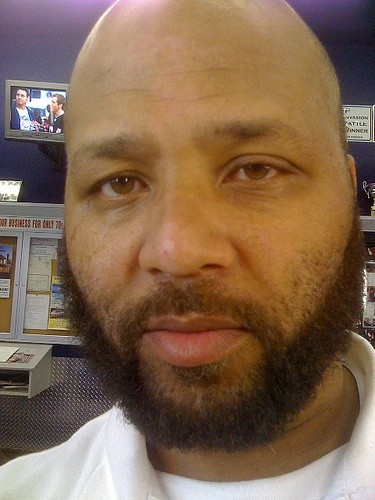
[0,0,375,500]
[10,88,66,134]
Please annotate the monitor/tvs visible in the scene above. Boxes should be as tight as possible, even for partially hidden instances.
[5,80,70,143]
